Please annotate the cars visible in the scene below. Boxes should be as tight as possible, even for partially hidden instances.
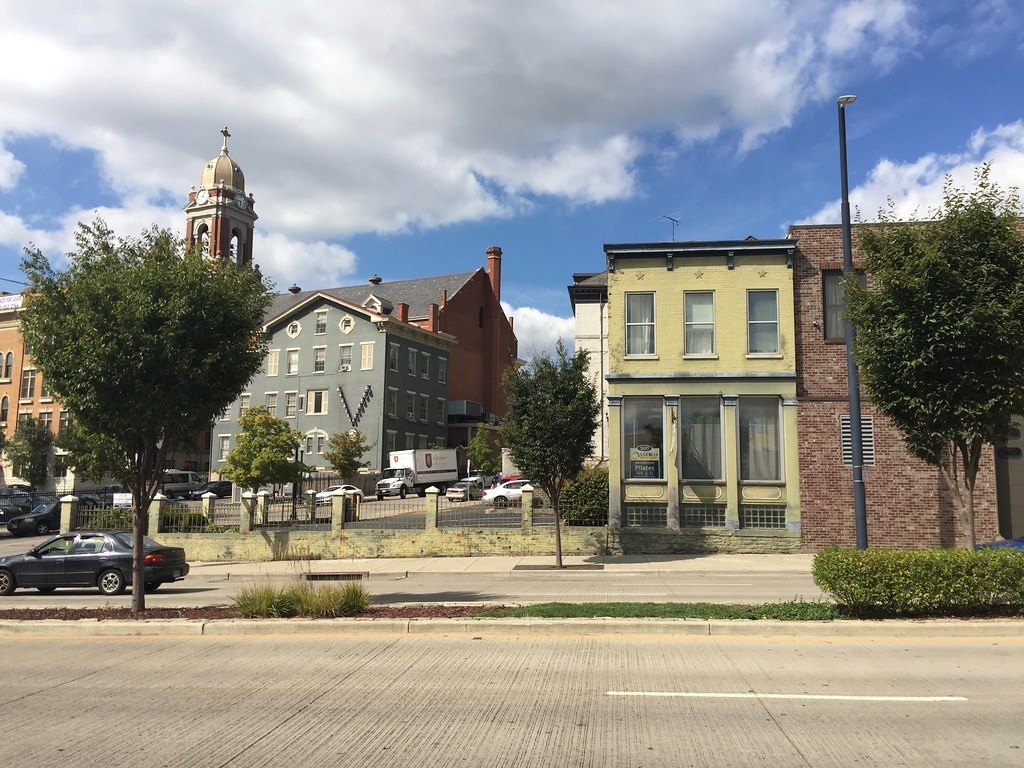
[0,530,190,596]
[482,480,531,508]
[461,469,495,489]
[80,484,135,507]
[316,484,365,507]
[446,482,482,502]
[188,481,232,501]
[7,503,62,536]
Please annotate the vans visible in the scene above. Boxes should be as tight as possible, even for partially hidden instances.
[146,470,207,500]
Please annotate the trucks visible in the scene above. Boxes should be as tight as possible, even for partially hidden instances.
[375,448,459,501]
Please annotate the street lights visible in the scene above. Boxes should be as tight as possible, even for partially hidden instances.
[836,94,867,545]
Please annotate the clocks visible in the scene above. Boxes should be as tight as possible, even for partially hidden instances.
[234,192,247,209]
[195,188,210,205]
